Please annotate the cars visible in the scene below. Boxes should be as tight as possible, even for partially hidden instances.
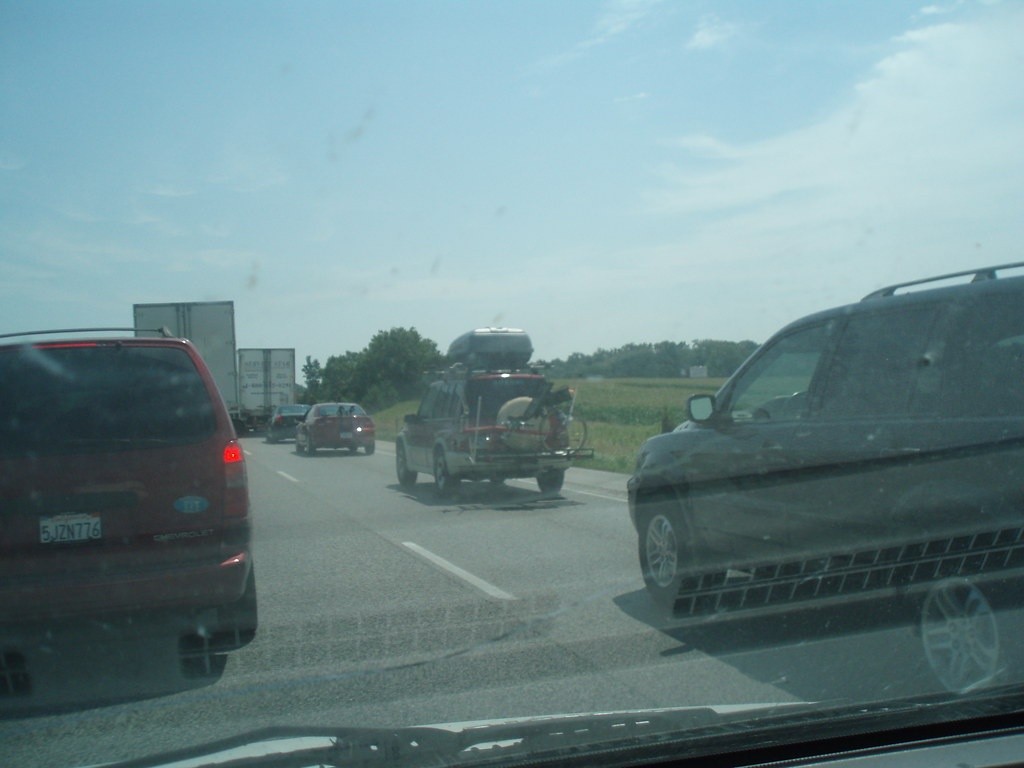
[294,402,377,456]
[266,403,311,443]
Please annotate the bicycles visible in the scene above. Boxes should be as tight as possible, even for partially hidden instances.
[500,379,587,457]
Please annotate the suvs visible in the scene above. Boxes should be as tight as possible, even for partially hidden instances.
[627,260,1024,613]
[396,326,595,504]
[0,326,258,678]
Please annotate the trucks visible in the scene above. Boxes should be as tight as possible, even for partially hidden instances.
[132,301,295,429]
